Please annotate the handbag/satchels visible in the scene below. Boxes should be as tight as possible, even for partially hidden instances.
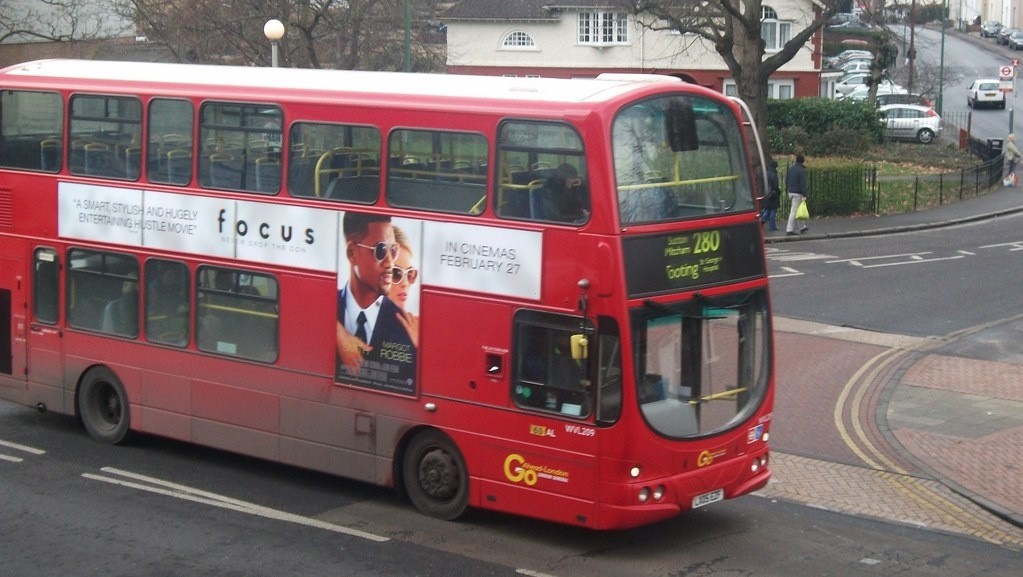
[761,188,780,209]
[1002,173,1018,188]
[795,197,809,220]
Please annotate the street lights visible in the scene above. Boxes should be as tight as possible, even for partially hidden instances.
[263,20,286,68]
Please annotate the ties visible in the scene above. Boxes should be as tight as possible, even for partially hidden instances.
[354,311,367,356]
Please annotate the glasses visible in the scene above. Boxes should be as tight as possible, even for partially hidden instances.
[392,266,418,285]
[356,241,400,263]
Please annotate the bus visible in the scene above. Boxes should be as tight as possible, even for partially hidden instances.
[1,56,786,533]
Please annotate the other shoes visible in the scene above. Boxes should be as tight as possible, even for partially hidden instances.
[769,227,779,231]
[786,232,798,235]
[801,228,808,233]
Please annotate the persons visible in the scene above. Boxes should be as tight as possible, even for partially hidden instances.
[758,162,781,230]
[538,163,589,222]
[335,211,419,378]
[118,271,139,336]
[784,155,809,236]
[531,331,595,389]
[1005,134,1023,175]
[889,41,899,67]
[907,45,916,60]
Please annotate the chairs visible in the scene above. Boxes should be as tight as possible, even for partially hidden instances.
[41,124,576,224]
[38,265,277,361]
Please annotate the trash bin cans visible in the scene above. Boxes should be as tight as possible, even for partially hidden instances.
[987,138,1003,158]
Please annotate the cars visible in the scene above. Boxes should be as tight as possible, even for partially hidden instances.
[823,50,932,110]
[997,26,1020,45]
[1008,32,1023,50]
[875,104,943,144]
[979,21,1004,38]
[967,79,1006,109]
[827,13,867,31]
